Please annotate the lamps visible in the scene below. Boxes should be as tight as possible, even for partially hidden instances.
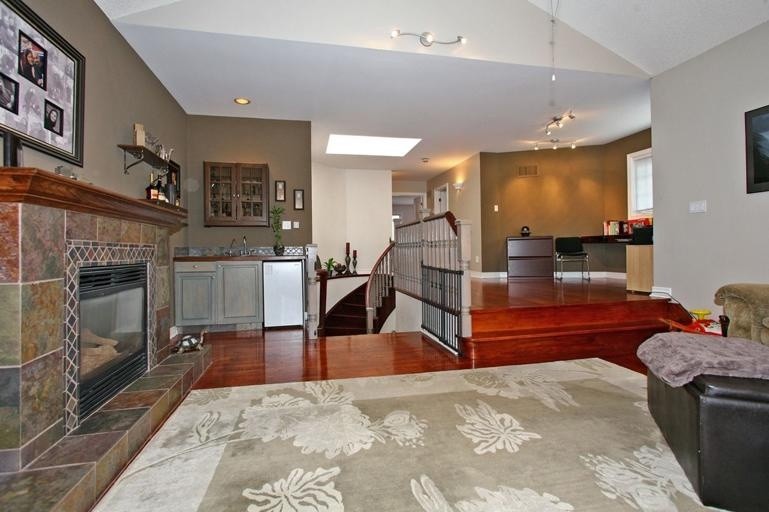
[533,140,575,151]
[387,29,465,49]
[494,205,498,211]
[544,110,575,135]
[452,177,463,190]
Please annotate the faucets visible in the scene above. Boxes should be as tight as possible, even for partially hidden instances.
[240,236,255,256]
[225,238,236,257]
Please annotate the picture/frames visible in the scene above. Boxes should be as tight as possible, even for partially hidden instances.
[274,181,286,201]
[745,105,769,194]
[293,188,303,210]
[0,0,86,168]
[516,162,540,178]
[166,160,180,198]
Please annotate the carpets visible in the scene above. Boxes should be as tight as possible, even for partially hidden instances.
[88,357,735,510]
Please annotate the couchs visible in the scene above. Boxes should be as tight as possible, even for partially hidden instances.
[635,284,768,511]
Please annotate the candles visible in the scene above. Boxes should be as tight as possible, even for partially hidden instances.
[343,243,351,258]
[352,250,357,259]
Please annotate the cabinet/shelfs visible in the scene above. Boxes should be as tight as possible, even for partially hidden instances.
[203,161,269,226]
[506,236,556,279]
[173,257,306,334]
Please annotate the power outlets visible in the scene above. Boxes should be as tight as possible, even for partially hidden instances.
[281,221,291,230]
[292,222,298,228]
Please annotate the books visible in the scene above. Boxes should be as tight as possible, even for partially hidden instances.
[603,218,653,236]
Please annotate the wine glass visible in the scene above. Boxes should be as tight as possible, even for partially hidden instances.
[145,137,161,155]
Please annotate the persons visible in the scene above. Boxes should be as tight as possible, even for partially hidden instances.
[46,109,59,130]
[23,49,42,83]
[31,51,44,85]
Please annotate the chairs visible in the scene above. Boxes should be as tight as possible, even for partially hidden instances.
[554,237,591,282]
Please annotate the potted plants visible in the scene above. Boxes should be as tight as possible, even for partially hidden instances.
[269,206,285,256]
[324,258,335,278]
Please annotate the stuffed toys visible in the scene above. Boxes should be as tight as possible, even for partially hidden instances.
[171,327,210,355]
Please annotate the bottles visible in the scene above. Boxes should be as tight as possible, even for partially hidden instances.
[146,170,158,200]
[158,175,166,201]
